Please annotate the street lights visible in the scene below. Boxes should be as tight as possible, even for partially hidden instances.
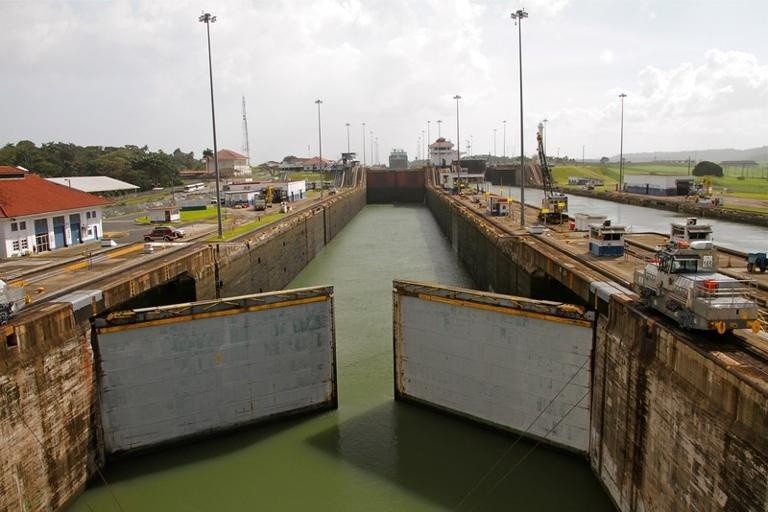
[199,12,224,238]
[315,99,326,198]
[64,178,71,188]
[511,9,530,226]
[453,94,462,196]
[618,93,629,191]
[417,120,442,160]
[346,122,379,168]
[493,118,548,160]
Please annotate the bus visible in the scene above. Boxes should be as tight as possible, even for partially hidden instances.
[183,182,205,192]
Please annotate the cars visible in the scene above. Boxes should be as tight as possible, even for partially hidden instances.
[143,225,186,242]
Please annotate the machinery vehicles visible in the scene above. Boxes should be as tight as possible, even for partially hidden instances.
[253,186,272,210]
[536,131,568,224]
[633,239,759,334]
